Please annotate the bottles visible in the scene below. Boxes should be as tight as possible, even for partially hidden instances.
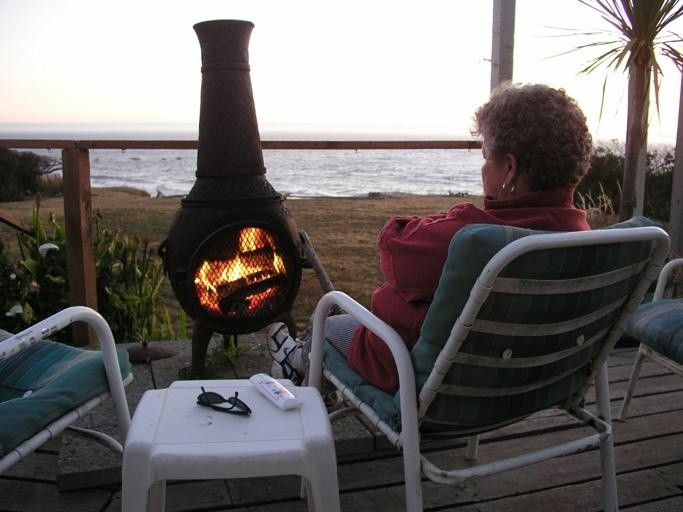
[251,374,297,410]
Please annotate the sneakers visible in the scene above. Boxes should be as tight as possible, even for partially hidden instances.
[268,322,307,386]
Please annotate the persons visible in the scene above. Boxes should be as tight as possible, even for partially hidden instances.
[266,82,593,410]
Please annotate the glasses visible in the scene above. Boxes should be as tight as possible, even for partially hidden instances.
[196,386,252,416]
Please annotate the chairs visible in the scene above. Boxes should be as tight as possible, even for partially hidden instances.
[0,301,135,512]
[303,210,671,511]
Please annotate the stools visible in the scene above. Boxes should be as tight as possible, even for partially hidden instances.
[119,375,343,512]
